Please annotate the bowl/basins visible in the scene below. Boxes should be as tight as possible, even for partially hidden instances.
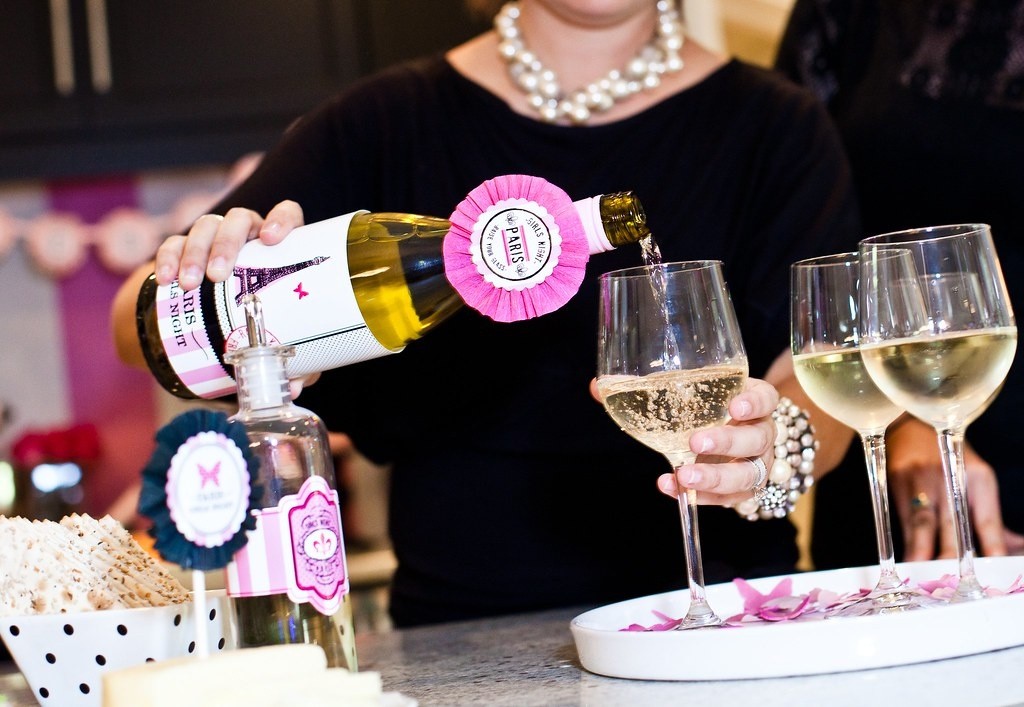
[0,591,217,707]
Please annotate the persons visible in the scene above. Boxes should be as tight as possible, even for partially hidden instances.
[151,0,866,629]
[772,0,1024,563]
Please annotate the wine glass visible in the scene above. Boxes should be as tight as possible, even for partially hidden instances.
[597,261,749,630]
[791,222,1017,621]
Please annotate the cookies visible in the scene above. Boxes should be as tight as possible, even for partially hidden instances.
[0,512,194,615]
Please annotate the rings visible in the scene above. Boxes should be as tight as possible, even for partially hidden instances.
[909,491,929,508]
[746,456,768,489]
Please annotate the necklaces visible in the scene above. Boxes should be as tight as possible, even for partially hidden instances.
[494,0,690,126]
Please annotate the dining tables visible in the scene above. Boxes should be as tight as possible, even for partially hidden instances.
[0,607,1024,707]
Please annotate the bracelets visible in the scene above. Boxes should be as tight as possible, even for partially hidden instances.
[737,396,821,521]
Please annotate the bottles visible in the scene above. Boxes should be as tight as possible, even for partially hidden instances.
[222,348,357,673]
[112,193,650,400]
